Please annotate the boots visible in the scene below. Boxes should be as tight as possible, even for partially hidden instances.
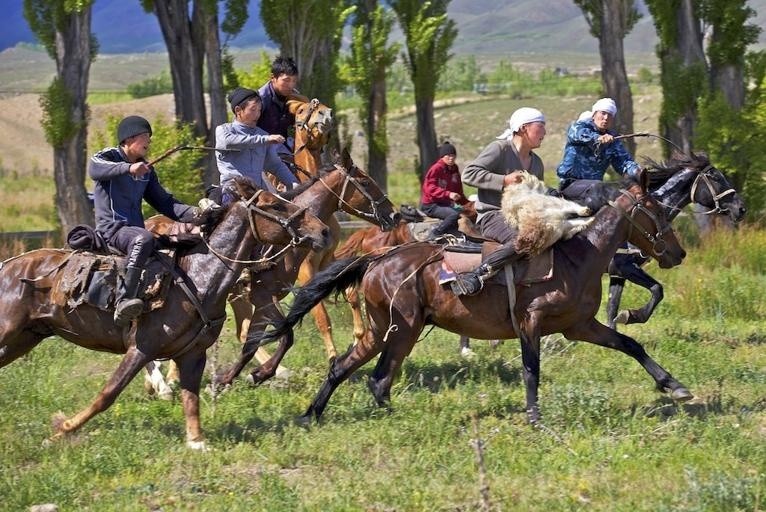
[113,266,144,328]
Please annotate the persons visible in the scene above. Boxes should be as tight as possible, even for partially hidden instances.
[419,141,470,240]
[88,116,205,326]
[450,106,546,297]
[557,97,642,197]
[214,86,301,279]
[253,56,301,158]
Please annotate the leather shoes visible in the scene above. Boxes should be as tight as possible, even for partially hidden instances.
[450,274,481,296]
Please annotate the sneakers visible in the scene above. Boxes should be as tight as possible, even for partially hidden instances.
[236,267,251,283]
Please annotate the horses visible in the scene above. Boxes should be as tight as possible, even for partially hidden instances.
[328,195,480,309]
[138,97,364,383]
[455,151,747,362]
[238,166,691,428]
[127,148,405,401]
[0,167,332,454]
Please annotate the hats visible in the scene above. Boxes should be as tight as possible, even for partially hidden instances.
[228,87,257,112]
[116,116,153,145]
[439,142,456,158]
